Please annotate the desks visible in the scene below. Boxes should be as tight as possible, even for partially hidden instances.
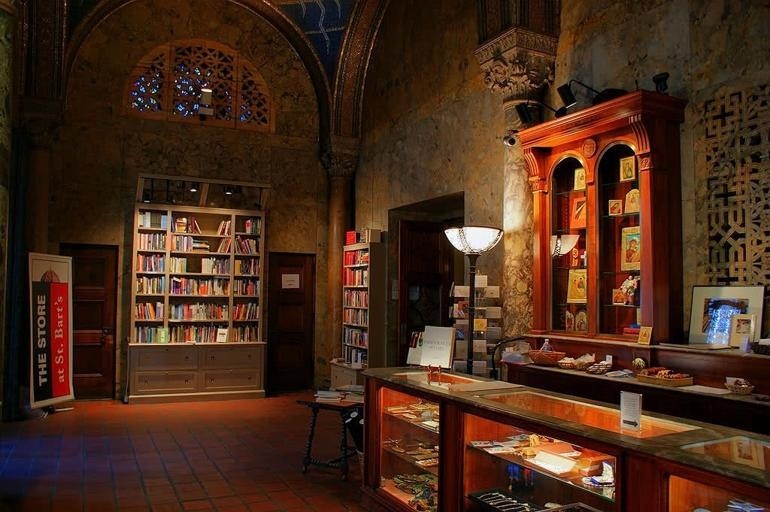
[295,395,363,481]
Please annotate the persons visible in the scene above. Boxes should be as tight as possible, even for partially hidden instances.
[623,163,632,178]
[570,277,585,299]
[610,203,621,214]
[619,274,638,305]
[626,239,640,263]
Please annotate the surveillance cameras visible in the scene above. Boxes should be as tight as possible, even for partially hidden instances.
[502,135,516,146]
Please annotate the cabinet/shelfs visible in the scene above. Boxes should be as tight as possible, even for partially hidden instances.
[359,363,740,512]
[517,90,686,343]
[127,202,269,403]
[329,242,388,391]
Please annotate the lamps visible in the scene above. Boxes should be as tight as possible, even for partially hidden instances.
[443,224,505,377]
[194,83,218,124]
[514,94,569,126]
[556,75,631,113]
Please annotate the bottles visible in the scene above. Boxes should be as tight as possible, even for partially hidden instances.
[542,339,550,350]
[634,280,640,306]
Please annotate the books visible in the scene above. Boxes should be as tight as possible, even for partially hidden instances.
[313,229,381,404]
[134,211,261,344]
[406,274,502,380]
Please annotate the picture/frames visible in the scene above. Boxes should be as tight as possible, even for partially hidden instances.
[607,155,642,272]
[687,281,767,344]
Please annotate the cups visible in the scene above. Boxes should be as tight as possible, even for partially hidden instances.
[758,338,770,345]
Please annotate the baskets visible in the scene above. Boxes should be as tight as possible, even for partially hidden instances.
[636,374,693,387]
[725,383,754,395]
[529,350,611,375]
